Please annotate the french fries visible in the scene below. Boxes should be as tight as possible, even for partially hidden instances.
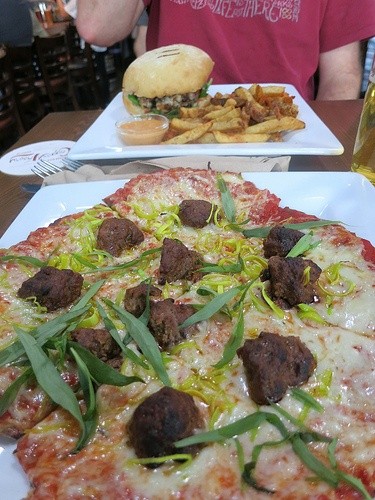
[160,85,306,142]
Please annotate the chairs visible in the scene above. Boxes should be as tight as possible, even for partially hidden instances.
[0,27,108,137]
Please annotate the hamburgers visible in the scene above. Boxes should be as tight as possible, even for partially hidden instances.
[123,44,215,119]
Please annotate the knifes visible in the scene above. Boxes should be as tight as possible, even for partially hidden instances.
[21,183,42,193]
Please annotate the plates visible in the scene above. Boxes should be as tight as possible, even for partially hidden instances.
[0,175,375,499]
[68,82,344,162]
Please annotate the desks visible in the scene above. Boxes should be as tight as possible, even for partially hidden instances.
[0,100,375,500]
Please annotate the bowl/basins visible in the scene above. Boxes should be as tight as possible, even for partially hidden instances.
[115,116,169,146]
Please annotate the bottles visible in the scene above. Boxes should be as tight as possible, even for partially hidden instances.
[349,53,374,186]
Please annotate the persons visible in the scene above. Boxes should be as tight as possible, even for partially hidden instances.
[362,36,375,99]
[134,11,148,58]
[0,0,108,57]
[74,0,375,102]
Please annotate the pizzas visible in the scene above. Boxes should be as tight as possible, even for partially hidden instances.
[0,168,375,500]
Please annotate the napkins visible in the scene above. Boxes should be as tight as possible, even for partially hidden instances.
[39,155,291,190]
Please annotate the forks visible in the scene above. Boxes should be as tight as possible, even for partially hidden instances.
[31,160,62,179]
[60,158,84,170]
